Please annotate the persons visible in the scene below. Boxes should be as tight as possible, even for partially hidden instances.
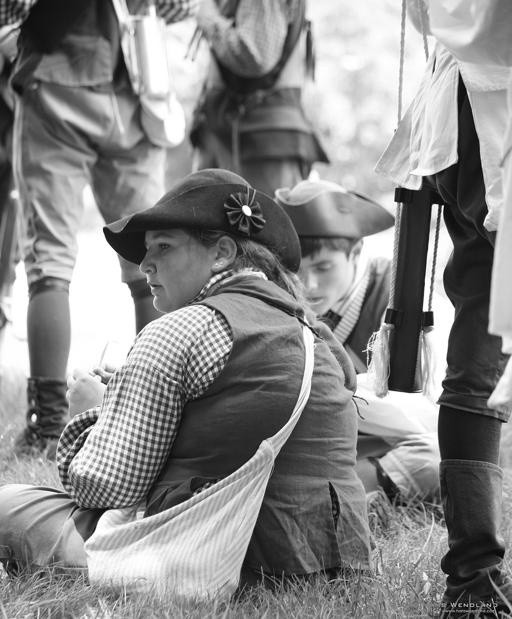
[0,1,511,619]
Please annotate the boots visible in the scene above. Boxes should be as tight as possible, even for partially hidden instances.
[19,376,69,460]
[438,458,512,619]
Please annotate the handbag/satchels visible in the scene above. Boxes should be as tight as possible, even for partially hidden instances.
[83,445,274,601]
[124,15,214,150]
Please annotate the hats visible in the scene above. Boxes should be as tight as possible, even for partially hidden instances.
[103,166,301,273]
[275,178,395,240]
